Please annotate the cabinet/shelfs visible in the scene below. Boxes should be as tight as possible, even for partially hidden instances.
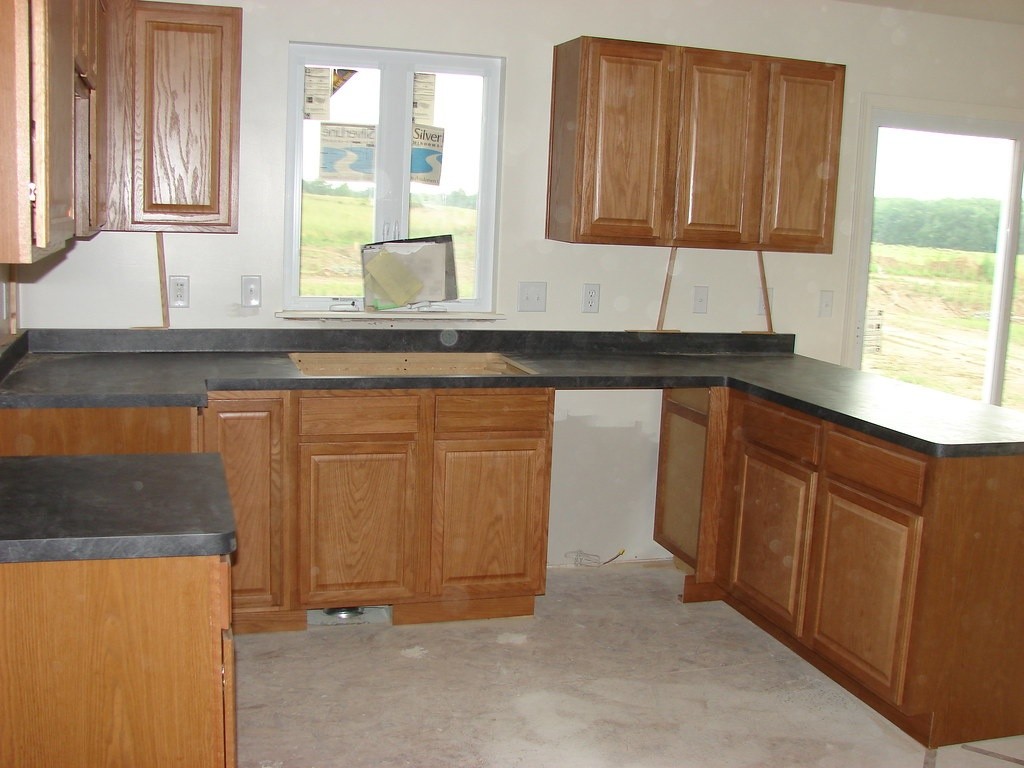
[549,35,846,254]
[74,0,106,238]
[106,0,242,233]
[293,386,554,627]
[0,0,75,265]
[72,0,97,100]
[200,388,294,634]
[715,387,1023,750]
[0,552,238,768]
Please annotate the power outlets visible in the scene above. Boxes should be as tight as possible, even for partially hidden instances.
[168,275,190,308]
[756,288,773,316]
[581,283,600,313]
